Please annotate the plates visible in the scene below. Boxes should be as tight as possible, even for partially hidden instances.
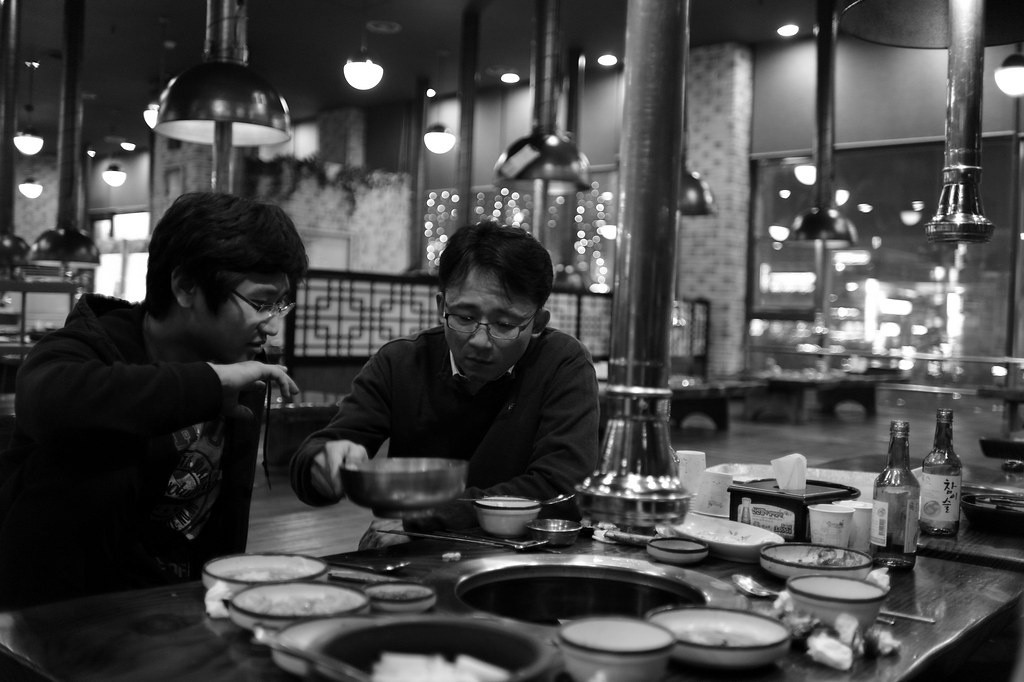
[642,604,792,668]
[759,542,873,580]
[671,512,786,562]
[270,615,376,676]
[645,537,710,564]
[200,551,328,601]
[359,580,437,614]
[226,579,371,635]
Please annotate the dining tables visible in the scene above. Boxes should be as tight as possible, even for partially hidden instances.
[0,456,1024,682]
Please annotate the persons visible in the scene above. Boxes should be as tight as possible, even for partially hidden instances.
[288,220,600,552]
[0,192,310,608]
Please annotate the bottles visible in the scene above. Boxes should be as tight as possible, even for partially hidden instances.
[869,419,920,574]
[920,407,963,538]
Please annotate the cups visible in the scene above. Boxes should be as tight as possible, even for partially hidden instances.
[554,616,679,682]
[806,499,873,554]
[676,450,733,520]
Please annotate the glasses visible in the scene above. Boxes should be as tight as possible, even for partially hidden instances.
[234,290,297,319]
[443,302,537,340]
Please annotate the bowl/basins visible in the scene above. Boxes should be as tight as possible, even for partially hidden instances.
[523,518,584,547]
[338,457,470,520]
[960,493,1024,531]
[786,572,888,631]
[306,614,553,682]
[472,496,542,536]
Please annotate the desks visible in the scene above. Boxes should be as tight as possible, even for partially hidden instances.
[592,374,747,436]
[743,372,909,421]
[252,402,344,462]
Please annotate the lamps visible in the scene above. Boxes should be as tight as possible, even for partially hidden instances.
[18,178,42,199]
[14,57,43,155]
[344,31,384,90]
[424,50,455,153]
[994,51,1024,98]
[103,166,126,186]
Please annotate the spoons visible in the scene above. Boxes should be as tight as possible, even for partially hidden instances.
[432,529,550,551]
[731,574,936,624]
[326,559,412,574]
[457,491,577,505]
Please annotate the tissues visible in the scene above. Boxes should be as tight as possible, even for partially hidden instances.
[727,453,861,543]
